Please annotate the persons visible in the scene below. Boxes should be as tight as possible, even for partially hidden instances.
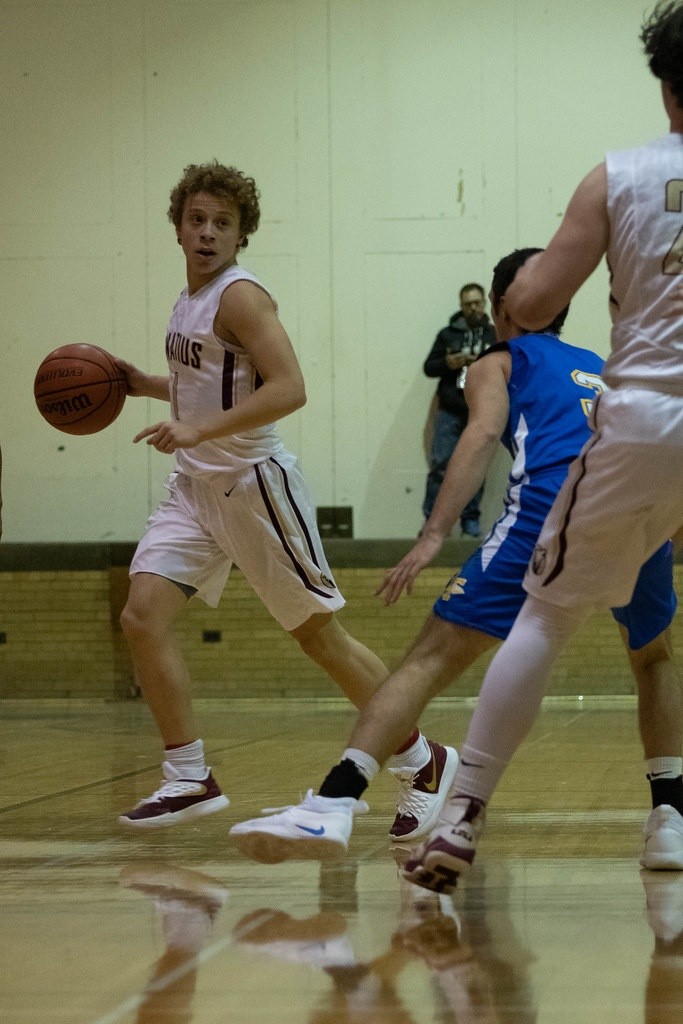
[120,861,682,1024]
[230,243,683,876]
[418,281,496,539]
[395,1,682,897]
[115,160,460,845]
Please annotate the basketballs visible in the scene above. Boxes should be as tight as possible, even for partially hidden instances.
[31,339,130,440]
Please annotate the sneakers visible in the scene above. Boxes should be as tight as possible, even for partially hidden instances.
[117,861,230,921]
[228,788,369,864]
[639,804,683,871]
[234,908,355,968]
[388,735,458,842]
[116,760,230,828]
[400,795,486,895]
[389,842,456,923]
[641,871,683,941]
[391,916,474,968]
[318,858,361,919]
